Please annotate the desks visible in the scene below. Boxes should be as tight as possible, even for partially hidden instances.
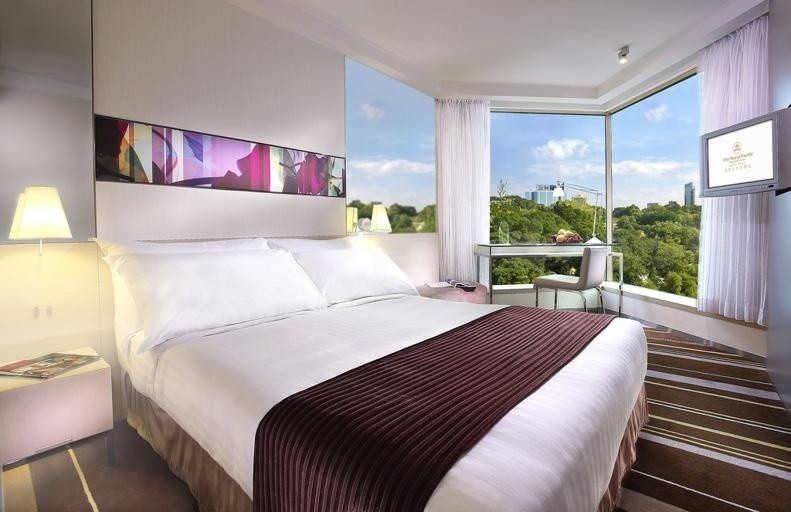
[472,241,624,316]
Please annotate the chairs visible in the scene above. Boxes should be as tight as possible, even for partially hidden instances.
[534,246,608,314]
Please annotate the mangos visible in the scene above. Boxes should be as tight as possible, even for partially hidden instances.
[556,235,565,242]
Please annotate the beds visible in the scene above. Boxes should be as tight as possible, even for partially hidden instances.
[102,230,646,512]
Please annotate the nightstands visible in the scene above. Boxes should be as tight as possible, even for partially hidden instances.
[419,278,488,305]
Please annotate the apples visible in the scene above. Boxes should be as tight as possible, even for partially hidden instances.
[565,231,573,235]
[557,229,565,235]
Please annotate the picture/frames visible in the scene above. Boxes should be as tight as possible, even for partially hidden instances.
[93,112,347,198]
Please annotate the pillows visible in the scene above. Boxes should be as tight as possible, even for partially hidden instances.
[103,235,420,352]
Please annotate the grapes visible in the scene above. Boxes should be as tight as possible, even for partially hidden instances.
[565,233,582,242]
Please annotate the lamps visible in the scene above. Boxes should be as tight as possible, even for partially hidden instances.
[347,203,393,233]
[7,184,74,248]
[553,179,604,244]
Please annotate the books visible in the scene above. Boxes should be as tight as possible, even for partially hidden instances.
[1,351,102,379]
[424,279,476,289]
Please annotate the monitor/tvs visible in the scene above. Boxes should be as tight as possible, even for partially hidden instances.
[698,106,791,198]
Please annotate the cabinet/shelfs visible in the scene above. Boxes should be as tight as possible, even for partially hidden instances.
[0,347,115,468]
